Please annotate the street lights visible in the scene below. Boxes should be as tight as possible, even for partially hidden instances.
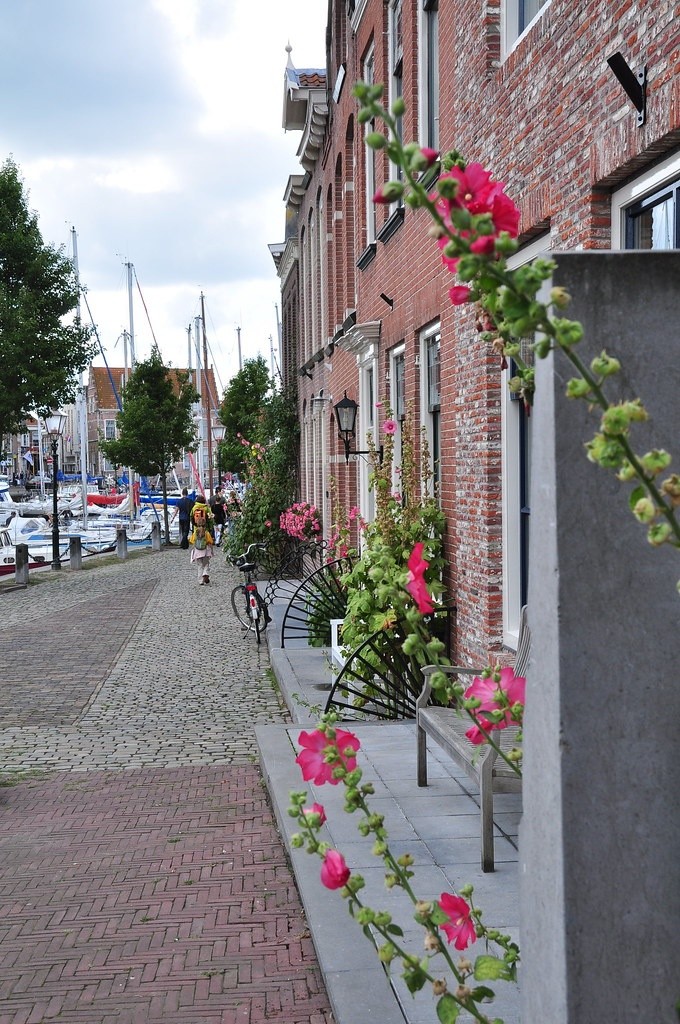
[210,418,227,485]
[43,410,69,570]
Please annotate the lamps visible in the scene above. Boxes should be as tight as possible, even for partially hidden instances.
[334,389,384,472]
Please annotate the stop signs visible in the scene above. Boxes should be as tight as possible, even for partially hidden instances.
[46,458,52,464]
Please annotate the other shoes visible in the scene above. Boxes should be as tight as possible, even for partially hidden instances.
[203,575,209,583]
[217,544,220,547]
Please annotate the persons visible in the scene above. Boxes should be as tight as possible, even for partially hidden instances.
[189,517,213,585]
[151,478,155,491]
[171,486,242,550]
[15,469,31,486]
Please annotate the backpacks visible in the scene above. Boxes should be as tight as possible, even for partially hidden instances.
[195,528,207,550]
[193,506,208,526]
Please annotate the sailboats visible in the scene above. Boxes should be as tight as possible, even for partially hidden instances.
[0,226,282,575]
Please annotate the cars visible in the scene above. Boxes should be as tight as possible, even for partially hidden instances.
[25,476,54,490]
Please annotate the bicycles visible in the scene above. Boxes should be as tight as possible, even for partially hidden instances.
[226,543,272,645]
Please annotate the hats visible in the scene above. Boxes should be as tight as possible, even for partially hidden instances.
[197,495,205,501]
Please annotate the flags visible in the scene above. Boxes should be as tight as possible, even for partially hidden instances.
[67,435,71,441]
[24,450,34,466]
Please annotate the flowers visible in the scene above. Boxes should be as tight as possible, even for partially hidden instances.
[279,502,322,546]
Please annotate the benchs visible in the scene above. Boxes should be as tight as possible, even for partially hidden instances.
[415,603,533,875]
[330,619,413,719]
